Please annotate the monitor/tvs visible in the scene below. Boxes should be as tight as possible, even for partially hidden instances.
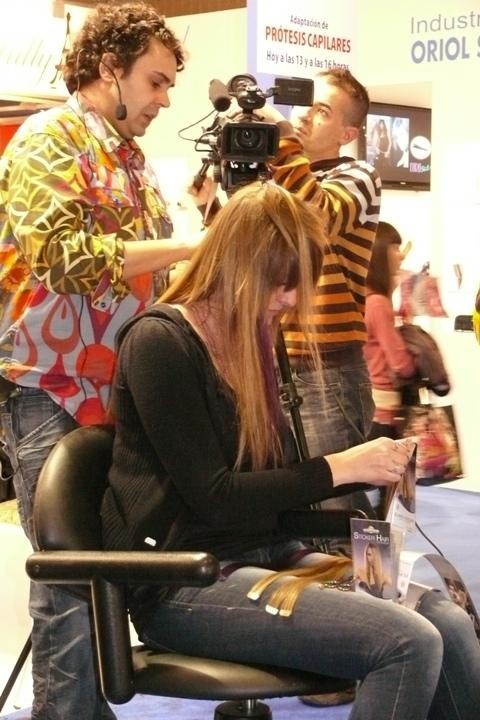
[358,101,432,191]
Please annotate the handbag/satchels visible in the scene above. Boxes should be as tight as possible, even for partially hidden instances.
[398,405,465,486]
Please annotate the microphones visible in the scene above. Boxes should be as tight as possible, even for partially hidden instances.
[97,58,127,120]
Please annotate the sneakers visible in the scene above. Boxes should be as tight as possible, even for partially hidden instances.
[300,686,355,706]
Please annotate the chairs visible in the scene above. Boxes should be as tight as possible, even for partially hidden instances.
[22,425,360,719]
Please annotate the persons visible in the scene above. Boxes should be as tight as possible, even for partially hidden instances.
[362,220,418,441]
[356,542,386,599]
[97,180,480,720]
[0,2,208,720]
[186,65,382,557]
[375,119,392,167]
